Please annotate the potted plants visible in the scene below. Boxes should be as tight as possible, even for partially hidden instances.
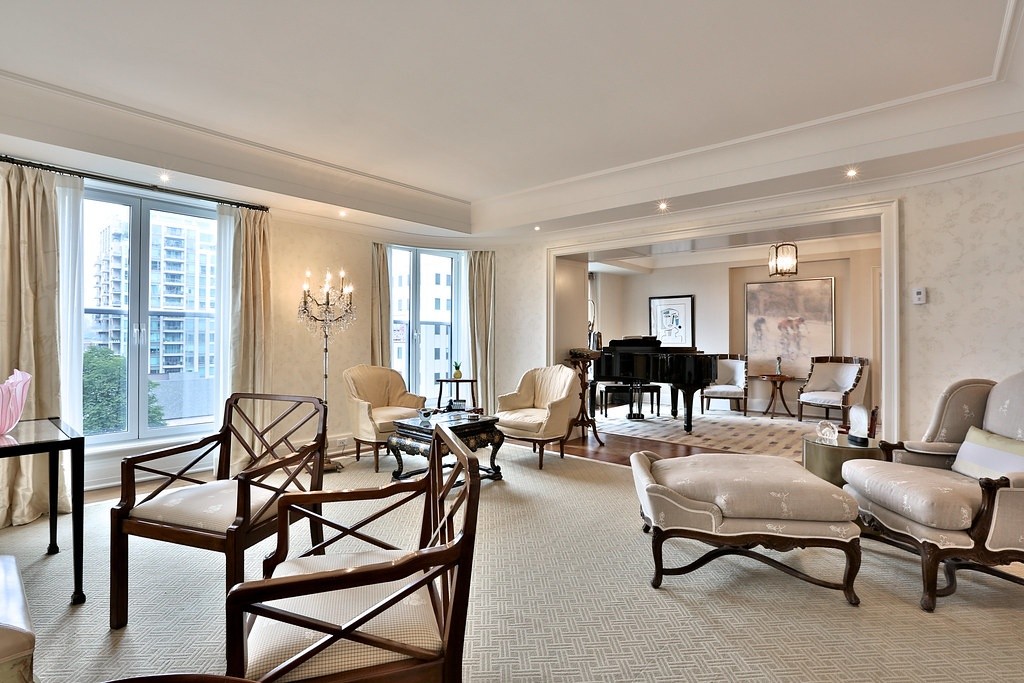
[454,361,462,379]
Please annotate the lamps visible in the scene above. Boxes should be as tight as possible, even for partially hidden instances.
[768,241,798,278]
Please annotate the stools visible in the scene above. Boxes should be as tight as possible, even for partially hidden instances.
[599,383,661,418]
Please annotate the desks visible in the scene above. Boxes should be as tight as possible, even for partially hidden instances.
[759,374,795,419]
[800,433,886,535]
[0,417,86,605]
[386,411,505,488]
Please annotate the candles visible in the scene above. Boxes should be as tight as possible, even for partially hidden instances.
[299,268,352,306]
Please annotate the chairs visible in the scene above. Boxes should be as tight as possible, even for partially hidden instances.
[701,354,869,426]
[227,423,480,683]
[110,392,327,630]
[493,364,577,469]
[343,364,426,473]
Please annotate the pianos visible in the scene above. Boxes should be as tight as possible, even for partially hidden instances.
[588,335,720,435]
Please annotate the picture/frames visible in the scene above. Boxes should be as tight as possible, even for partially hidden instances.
[649,295,695,347]
[744,277,836,381]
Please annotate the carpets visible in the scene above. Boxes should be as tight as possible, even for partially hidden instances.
[0,442,1024,683]
[588,402,882,462]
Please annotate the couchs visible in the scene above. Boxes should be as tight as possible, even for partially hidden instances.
[630,373,1024,612]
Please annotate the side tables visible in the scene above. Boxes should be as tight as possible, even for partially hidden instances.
[434,378,483,415]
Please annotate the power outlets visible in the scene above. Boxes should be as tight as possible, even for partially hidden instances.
[337,438,347,447]
